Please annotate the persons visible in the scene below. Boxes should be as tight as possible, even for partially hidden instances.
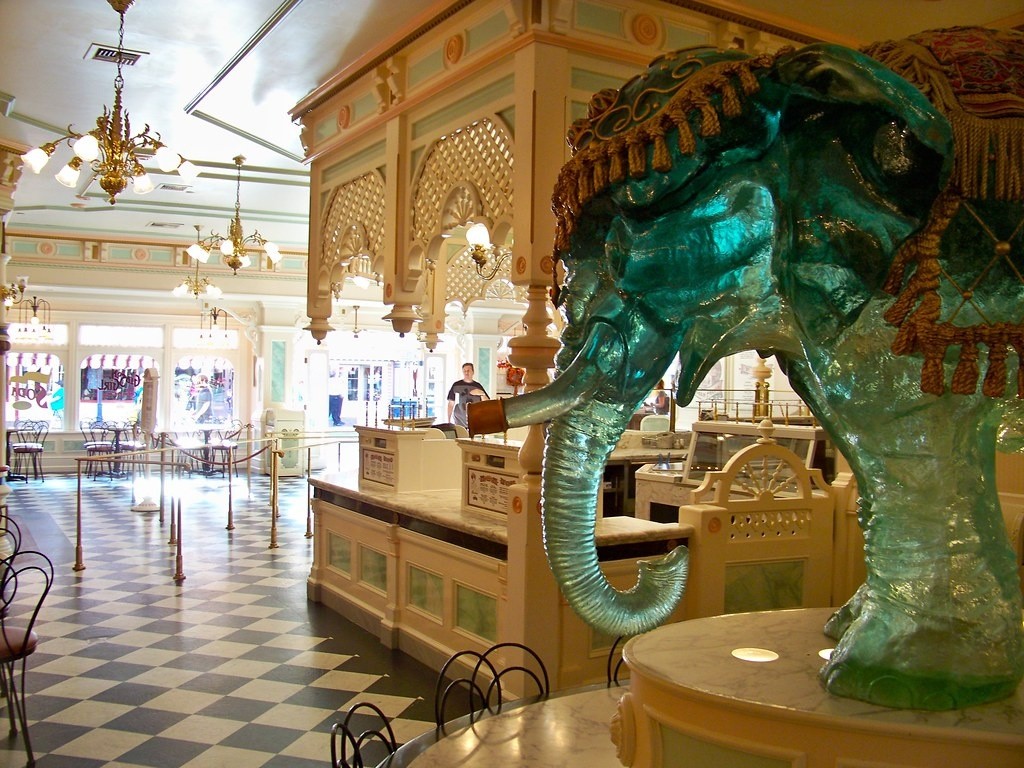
[187,374,213,475]
[650,380,668,415]
[447,363,490,430]
[48,380,64,428]
[327,370,346,426]
[134,378,143,427]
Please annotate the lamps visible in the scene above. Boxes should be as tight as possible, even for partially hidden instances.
[170,224,224,301]
[19,1,203,206]
[185,154,284,276]
[0,276,51,333]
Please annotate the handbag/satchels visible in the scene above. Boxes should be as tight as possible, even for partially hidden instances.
[656,391,669,412]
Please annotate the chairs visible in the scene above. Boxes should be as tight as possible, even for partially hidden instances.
[329,641,549,768]
[0,417,246,768]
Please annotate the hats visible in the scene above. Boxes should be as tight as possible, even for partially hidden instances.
[55,381,63,387]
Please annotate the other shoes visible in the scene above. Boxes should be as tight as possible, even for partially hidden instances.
[334,421,344,426]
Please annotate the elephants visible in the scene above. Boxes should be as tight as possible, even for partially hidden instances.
[466,19,1024,714]
